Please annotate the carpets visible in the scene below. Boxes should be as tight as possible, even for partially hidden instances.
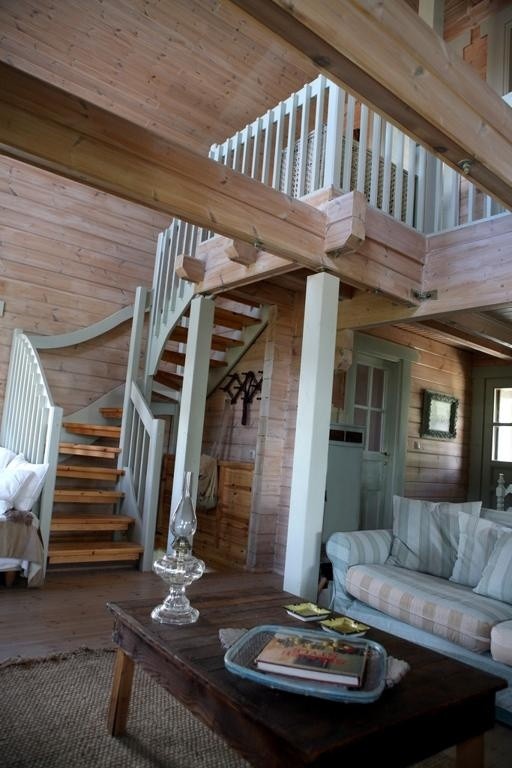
[1,645,458,768]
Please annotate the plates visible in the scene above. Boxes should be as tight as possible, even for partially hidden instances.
[282,602,371,639]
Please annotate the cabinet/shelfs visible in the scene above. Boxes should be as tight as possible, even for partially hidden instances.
[156,454,255,564]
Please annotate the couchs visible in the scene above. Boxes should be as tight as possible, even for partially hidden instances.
[325,505,511,728]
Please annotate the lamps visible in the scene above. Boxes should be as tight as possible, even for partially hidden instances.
[150,470,208,627]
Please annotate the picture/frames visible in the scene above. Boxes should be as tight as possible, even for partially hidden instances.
[421,389,459,439]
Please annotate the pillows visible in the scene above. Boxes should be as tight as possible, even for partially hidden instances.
[388,494,511,604]
[0,446,50,513]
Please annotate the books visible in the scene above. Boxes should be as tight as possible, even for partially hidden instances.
[256,629,369,687]
